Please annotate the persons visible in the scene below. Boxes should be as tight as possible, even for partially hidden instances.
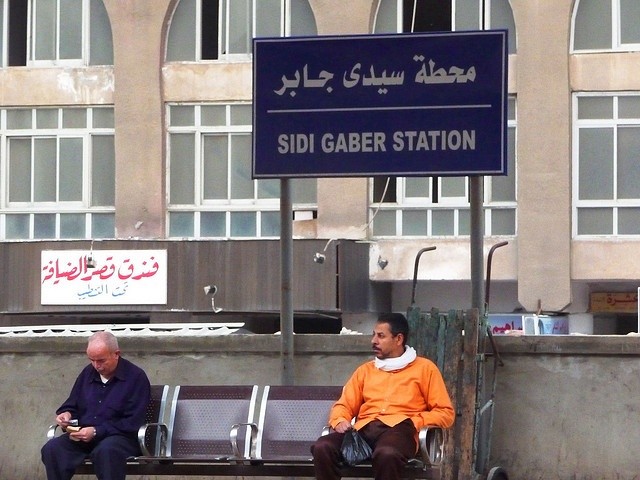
[310,312,455,480]
[40,332,150,479]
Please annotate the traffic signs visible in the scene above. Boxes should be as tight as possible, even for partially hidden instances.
[251,28,510,178]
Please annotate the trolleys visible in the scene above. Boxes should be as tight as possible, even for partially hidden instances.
[391,242,510,479]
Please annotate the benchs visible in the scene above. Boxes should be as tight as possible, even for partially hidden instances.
[47,384,444,479]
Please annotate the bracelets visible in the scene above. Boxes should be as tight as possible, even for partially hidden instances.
[92,426,97,439]
